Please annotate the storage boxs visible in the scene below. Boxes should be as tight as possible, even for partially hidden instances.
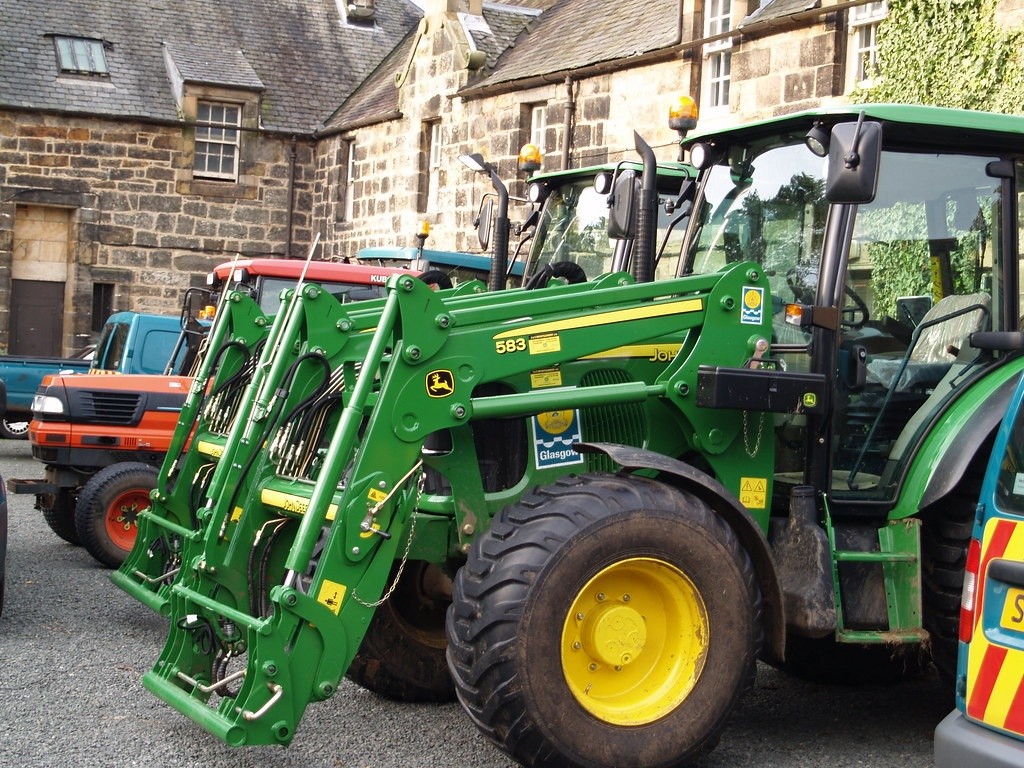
[696,358,826,417]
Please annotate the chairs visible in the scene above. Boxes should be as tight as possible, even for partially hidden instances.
[863,292,990,396]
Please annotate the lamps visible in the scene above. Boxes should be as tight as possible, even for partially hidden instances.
[459,152,498,178]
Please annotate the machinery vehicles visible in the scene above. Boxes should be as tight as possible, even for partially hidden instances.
[7,94,1024,767]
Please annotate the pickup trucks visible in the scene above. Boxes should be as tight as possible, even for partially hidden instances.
[0,310,214,440]
